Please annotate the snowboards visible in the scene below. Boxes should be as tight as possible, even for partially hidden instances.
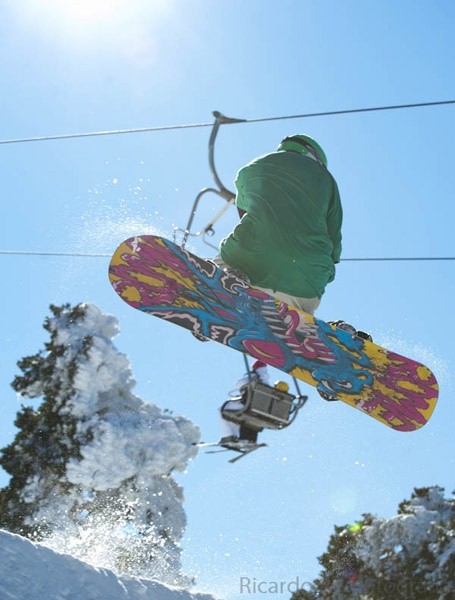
[108,235,438,431]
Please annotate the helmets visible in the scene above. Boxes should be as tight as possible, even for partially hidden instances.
[277,134,327,167]
[251,360,267,374]
[274,381,289,393]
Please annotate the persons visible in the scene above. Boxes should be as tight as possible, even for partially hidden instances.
[208,135,345,448]
